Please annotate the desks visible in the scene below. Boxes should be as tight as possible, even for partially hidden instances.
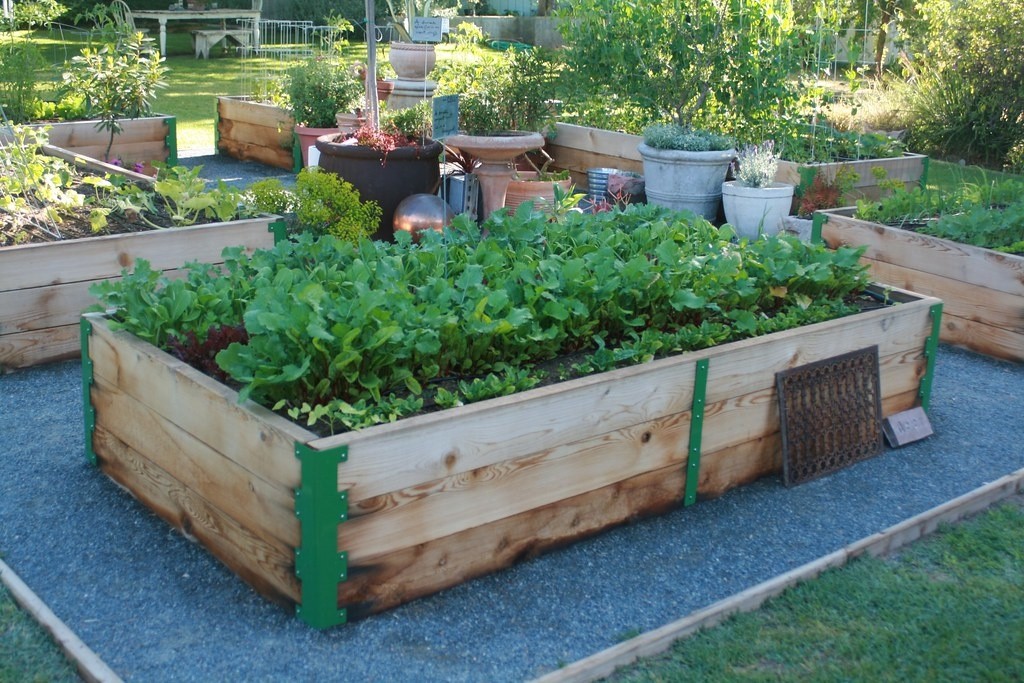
[124,8,262,58]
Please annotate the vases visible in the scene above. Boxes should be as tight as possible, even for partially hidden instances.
[721,178,795,247]
[606,171,647,214]
[586,167,621,203]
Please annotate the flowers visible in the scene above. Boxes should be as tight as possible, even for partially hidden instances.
[729,139,785,189]
[107,154,147,175]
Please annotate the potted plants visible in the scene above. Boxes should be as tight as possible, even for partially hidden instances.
[636,121,739,228]
[783,170,844,243]
[0,95,179,182]
[213,91,308,177]
[372,59,396,101]
[535,110,933,219]
[313,100,444,246]
[460,42,573,219]
[435,138,484,217]
[56,28,174,164]
[808,157,1024,367]
[0,120,285,377]
[444,91,546,242]
[78,199,947,631]
[374,0,462,82]
[277,47,366,171]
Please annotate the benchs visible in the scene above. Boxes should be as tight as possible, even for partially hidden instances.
[113,27,152,52]
[191,28,252,61]
